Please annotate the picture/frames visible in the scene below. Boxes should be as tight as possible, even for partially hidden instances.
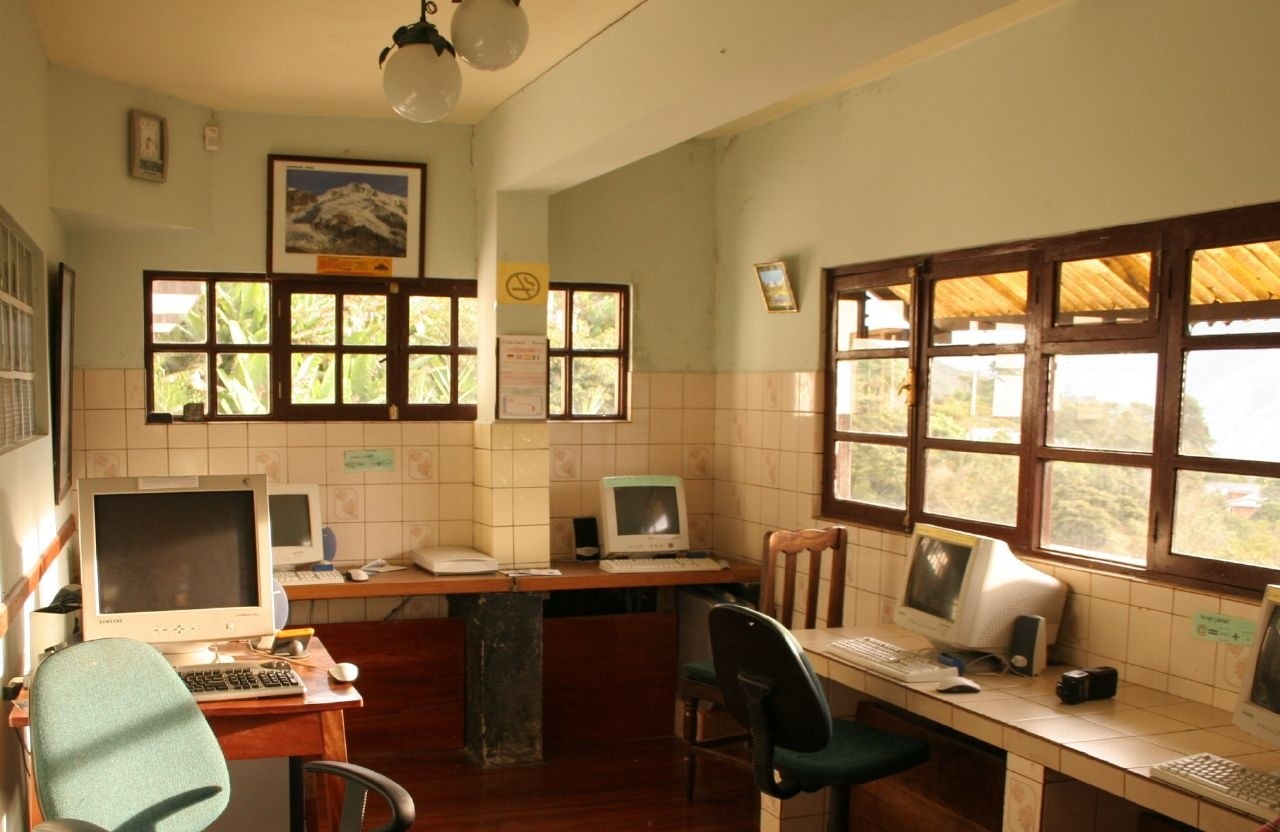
[752,261,800,314]
[267,152,428,283]
[52,258,78,507]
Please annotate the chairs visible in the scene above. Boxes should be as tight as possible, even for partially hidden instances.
[707,599,930,831]
[677,521,853,809]
[24,636,416,830]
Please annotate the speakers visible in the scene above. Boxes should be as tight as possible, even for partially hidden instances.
[1009,614,1048,677]
[571,517,599,564]
[311,527,336,570]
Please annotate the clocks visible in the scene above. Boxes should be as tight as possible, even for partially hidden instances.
[126,106,170,183]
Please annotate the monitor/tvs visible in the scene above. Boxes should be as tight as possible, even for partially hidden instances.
[267,483,323,571]
[1232,584,1279,748]
[598,476,689,558]
[76,474,275,663]
[893,523,1069,671]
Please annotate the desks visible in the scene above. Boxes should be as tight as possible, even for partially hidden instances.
[506,555,765,765]
[271,555,517,756]
[6,641,363,829]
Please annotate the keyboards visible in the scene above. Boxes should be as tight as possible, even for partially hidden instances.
[170,661,306,703]
[1148,752,1280,825]
[274,570,344,586]
[821,635,959,682]
[599,559,722,572]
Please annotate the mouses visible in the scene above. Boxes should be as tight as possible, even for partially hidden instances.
[718,560,730,569]
[935,675,982,692]
[329,662,360,684]
[346,569,368,582]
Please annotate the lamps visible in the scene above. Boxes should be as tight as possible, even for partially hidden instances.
[374,1,533,124]
